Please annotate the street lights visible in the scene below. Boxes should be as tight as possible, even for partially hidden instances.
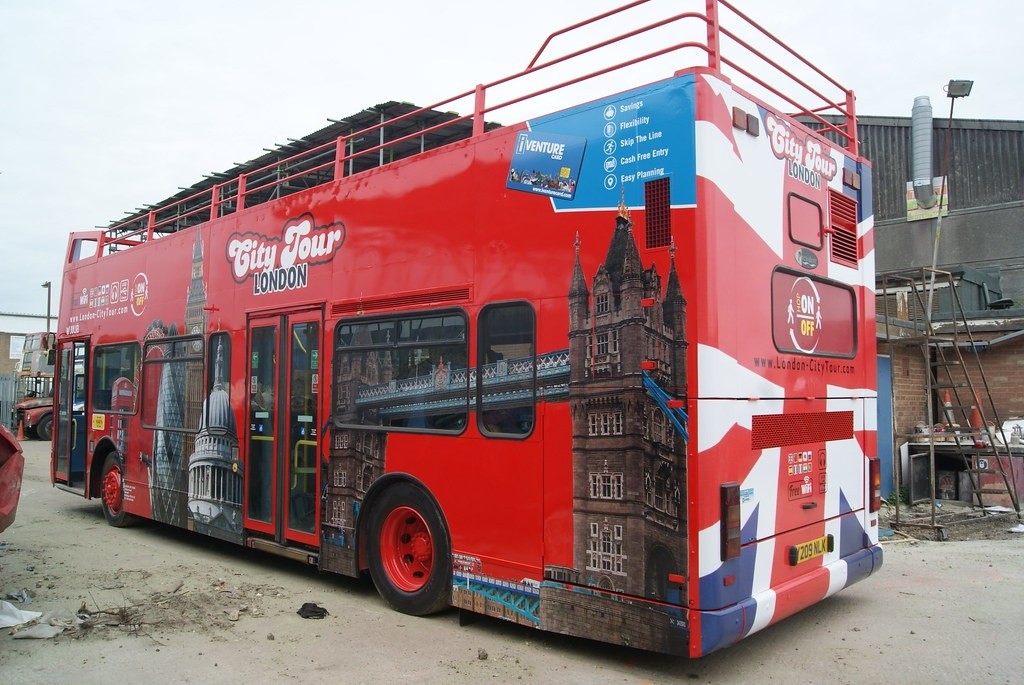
[923,79,974,335]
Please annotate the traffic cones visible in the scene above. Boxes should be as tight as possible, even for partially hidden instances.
[15,419,29,440]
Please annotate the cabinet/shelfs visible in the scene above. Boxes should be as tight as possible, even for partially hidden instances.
[899,441,1024,507]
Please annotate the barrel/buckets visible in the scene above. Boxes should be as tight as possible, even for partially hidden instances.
[914,422,960,442]
[958,471,973,502]
[981,421,995,438]
[938,471,956,499]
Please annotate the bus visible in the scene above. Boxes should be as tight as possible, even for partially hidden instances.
[49,0,884,661]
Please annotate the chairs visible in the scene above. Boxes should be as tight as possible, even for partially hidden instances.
[982,282,1015,310]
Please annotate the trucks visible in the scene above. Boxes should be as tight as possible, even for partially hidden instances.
[8,385,54,440]
